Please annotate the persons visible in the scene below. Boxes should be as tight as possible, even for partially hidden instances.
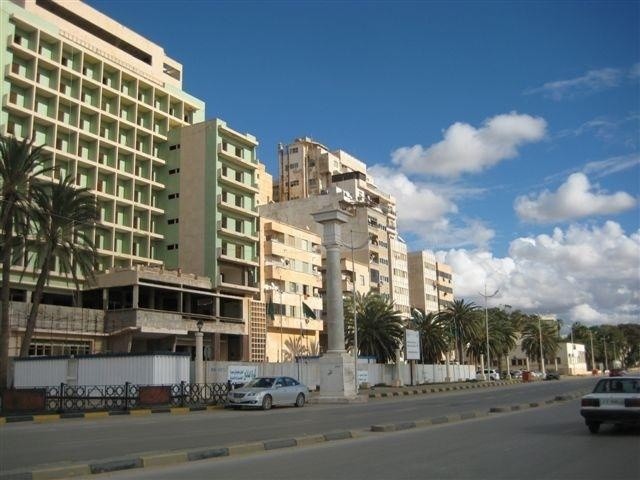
[614,382,625,392]
[602,380,616,392]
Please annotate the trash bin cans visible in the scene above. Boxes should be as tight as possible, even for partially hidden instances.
[522,371,531,382]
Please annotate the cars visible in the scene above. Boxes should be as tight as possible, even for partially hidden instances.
[227,376,308,411]
[512,370,522,378]
[477,369,500,381]
[580,376,639,433]
